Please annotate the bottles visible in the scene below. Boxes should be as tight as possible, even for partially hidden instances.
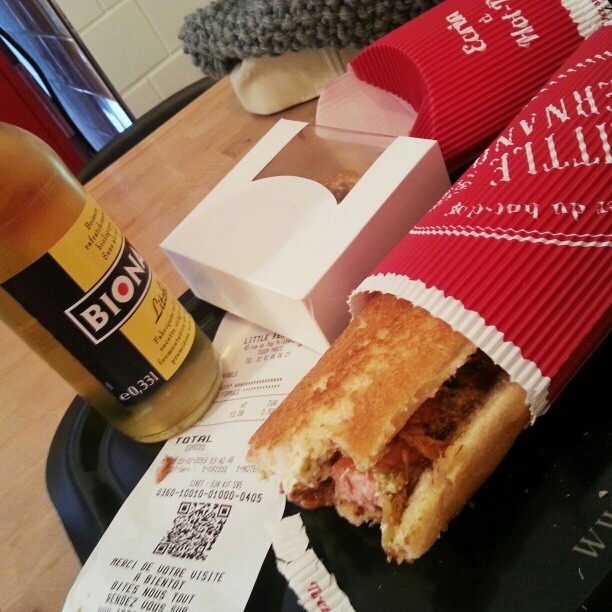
[0,119,225,443]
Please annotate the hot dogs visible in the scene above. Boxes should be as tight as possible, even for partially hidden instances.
[244,294,531,568]
